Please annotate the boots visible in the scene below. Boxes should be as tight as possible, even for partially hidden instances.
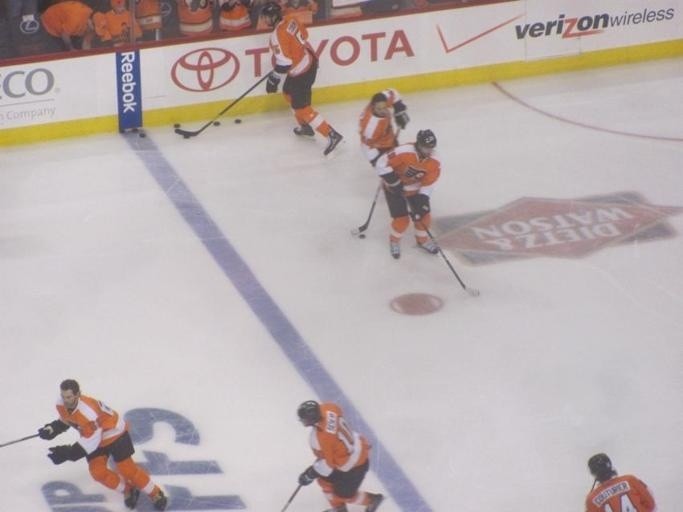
[414,233,439,255]
[292,121,315,138]
[146,483,169,511]
[389,232,403,259]
[364,493,385,512]
[322,125,347,159]
[117,480,140,509]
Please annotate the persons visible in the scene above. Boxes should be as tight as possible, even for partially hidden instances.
[298,400,383,512]
[39,380,167,512]
[260,5,343,157]
[5,0,319,54]
[359,88,410,168]
[587,454,655,512]
[375,130,441,260]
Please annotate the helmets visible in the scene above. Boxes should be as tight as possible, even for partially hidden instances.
[416,128,437,151]
[296,400,320,426]
[261,3,281,18]
[587,453,612,476]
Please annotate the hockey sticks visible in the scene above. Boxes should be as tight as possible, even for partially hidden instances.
[349,126,401,234]
[174,65,276,137]
[407,197,480,297]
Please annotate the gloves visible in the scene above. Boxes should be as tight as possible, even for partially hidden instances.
[381,183,403,195]
[408,193,430,223]
[395,113,409,130]
[264,74,281,94]
[37,424,57,440]
[296,464,317,487]
[46,444,69,466]
